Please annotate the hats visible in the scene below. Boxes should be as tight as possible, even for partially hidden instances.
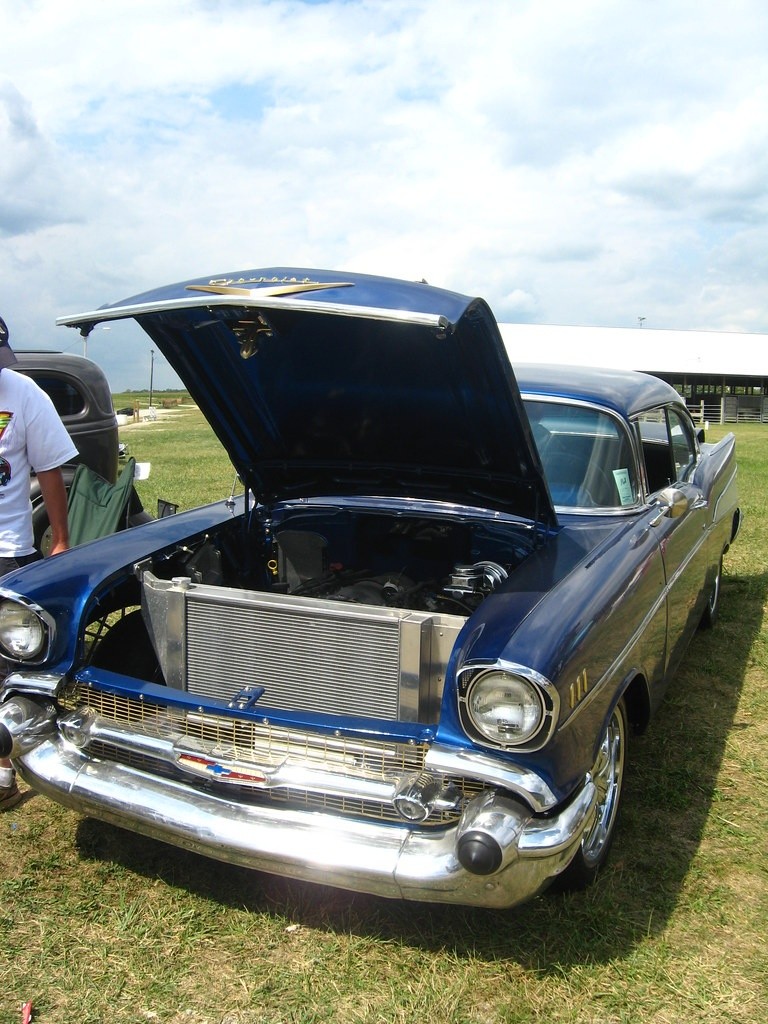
[0,316,19,372]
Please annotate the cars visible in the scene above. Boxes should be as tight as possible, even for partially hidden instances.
[0,266,741,912]
[2,344,180,566]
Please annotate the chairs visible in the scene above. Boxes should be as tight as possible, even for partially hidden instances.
[142,406,157,422]
[66,455,136,665]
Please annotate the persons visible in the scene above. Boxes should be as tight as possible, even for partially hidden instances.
[0,318,82,809]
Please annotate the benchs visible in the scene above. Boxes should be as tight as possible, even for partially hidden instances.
[547,435,673,506]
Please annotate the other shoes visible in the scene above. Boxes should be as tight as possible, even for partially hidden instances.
[0,767,21,809]
